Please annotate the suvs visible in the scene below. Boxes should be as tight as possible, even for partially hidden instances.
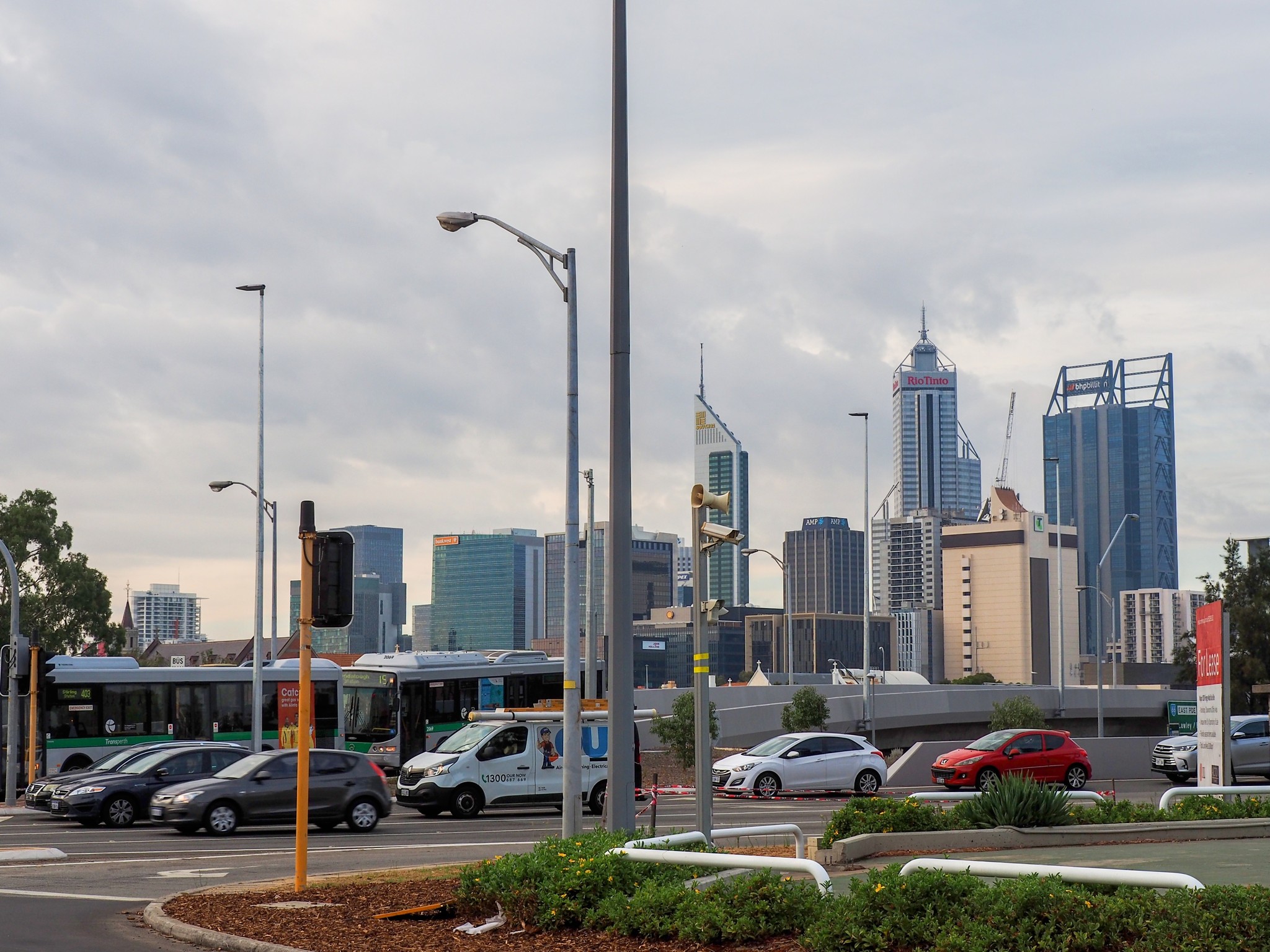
[1150,714,1270,783]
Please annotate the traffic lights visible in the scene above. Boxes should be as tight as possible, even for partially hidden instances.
[37,648,56,689]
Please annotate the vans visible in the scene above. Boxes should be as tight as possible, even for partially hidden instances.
[395,719,648,820]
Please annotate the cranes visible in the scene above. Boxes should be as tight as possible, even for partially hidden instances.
[994,391,1016,488]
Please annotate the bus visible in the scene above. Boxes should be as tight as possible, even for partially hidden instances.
[0,654,345,803]
[342,646,606,777]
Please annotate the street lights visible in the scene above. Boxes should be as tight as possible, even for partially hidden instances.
[435,209,583,842]
[740,548,795,685]
[849,412,873,731]
[209,478,279,660]
[1096,513,1140,738]
[1075,585,1117,689]
[236,283,266,756]
[1043,458,1067,718]
[879,646,885,684]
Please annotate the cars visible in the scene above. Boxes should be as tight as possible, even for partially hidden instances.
[931,728,1093,792]
[24,739,290,830]
[148,747,393,838]
[712,733,888,799]
